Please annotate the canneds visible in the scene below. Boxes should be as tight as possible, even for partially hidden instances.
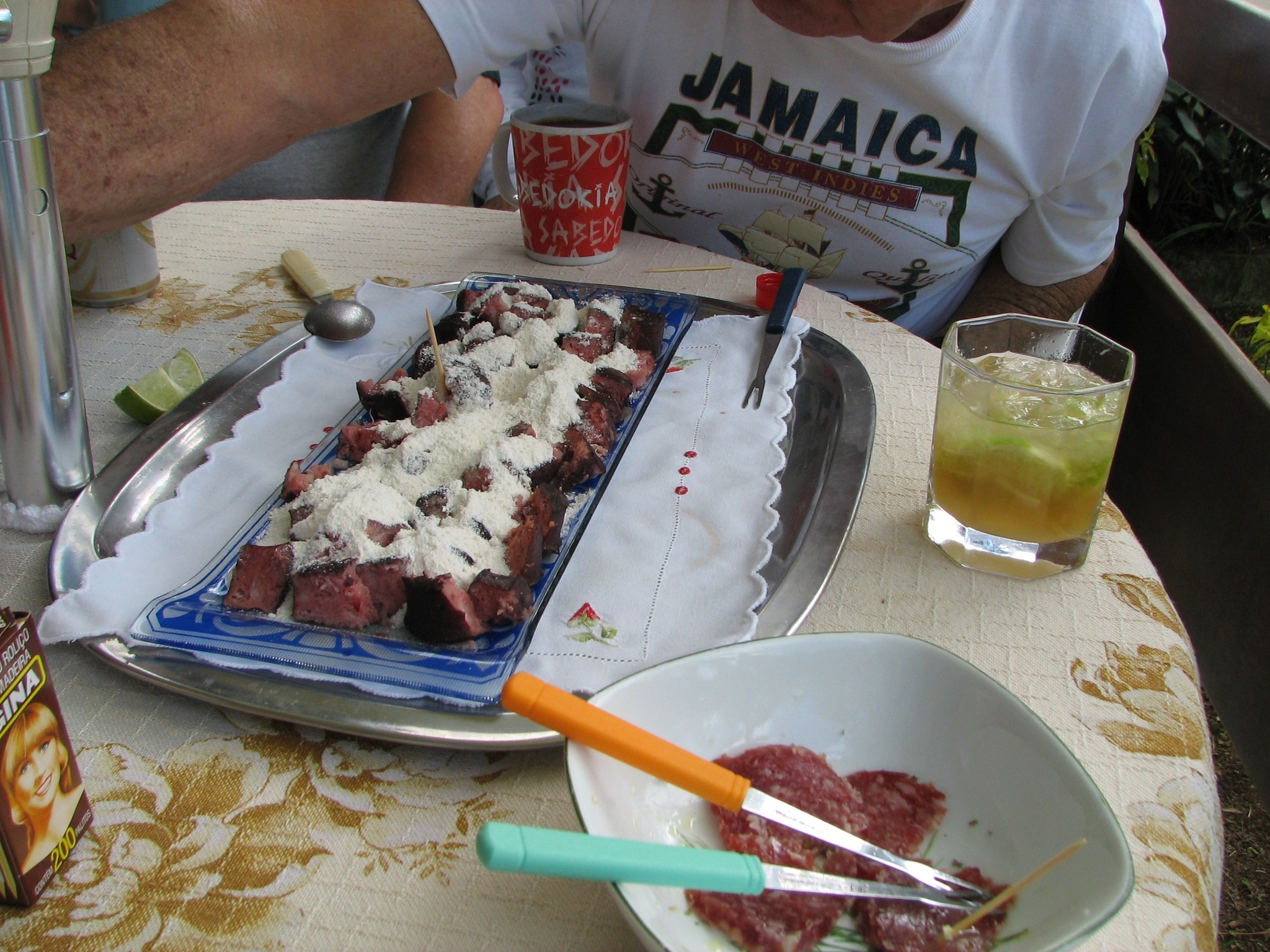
[65,219,160,309]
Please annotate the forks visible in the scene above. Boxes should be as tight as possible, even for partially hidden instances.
[477,673,994,913]
[743,267,807,409]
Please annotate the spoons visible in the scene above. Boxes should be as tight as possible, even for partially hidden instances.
[281,249,376,341]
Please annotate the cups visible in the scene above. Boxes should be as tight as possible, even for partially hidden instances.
[924,314,1135,580]
[492,103,634,266]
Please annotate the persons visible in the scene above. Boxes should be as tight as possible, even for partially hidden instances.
[35,0,1168,365]
[1,703,84,876]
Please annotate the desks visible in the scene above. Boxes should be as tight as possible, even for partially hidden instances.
[0,197,1227,952]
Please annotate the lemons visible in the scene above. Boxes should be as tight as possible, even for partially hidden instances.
[115,347,204,422]
[946,438,1109,501]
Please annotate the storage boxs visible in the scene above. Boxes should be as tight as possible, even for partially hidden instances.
[0,605,93,912]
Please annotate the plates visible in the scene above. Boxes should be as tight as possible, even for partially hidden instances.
[129,271,703,714]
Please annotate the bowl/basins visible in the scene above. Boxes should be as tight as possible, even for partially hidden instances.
[566,631,1135,952]
